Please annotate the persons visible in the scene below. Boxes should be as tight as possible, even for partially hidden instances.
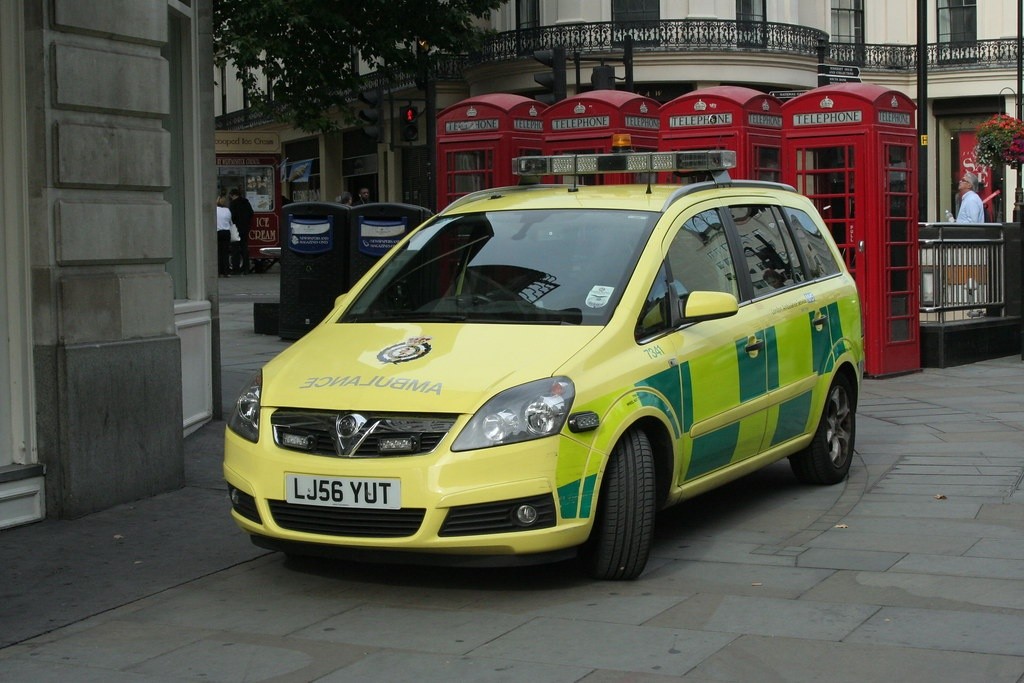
[338,187,370,207]
[217,188,255,277]
[956,173,985,223]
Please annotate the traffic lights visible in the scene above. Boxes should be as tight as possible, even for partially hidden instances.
[401,105,419,142]
[357,86,384,143]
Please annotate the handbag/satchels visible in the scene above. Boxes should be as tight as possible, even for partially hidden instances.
[229,220,241,242]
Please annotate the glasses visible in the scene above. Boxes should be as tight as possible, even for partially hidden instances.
[961,178,970,183]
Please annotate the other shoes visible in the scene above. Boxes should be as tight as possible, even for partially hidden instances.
[220,274,224,278]
[227,275,231,278]
[232,270,237,275]
[243,271,248,275]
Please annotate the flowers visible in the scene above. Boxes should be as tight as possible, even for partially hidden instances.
[977,114,1024,171]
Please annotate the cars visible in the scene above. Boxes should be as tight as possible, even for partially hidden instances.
[221,130,867,585]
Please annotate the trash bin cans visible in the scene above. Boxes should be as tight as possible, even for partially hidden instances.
[349,204,434,289]
[280,200,350,341]
